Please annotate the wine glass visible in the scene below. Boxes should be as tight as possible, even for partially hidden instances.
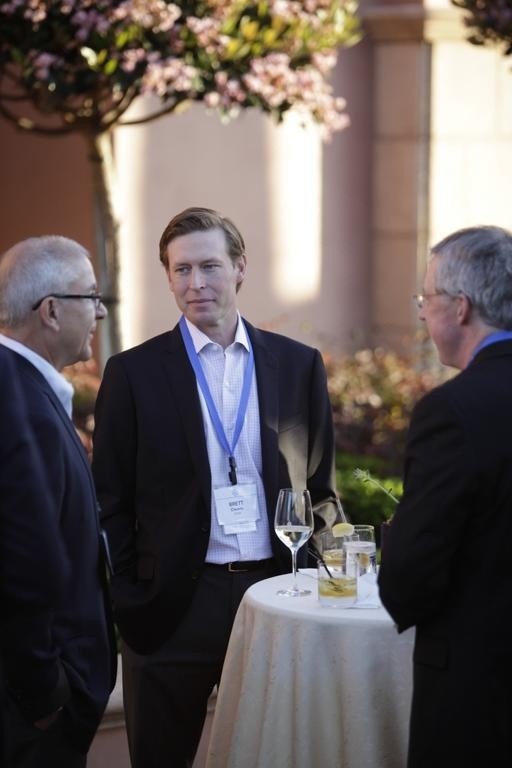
[273,488,313,599]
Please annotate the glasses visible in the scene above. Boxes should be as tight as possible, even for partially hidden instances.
[31,292,101,312]
[412,290,444,308]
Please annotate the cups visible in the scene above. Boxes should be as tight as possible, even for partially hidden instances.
[340,523,378,579]
[311,529,362,610]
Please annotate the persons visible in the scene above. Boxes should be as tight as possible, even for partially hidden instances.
[376,227,512,766]
[0,235,121,767]
[90,206,336,767]
